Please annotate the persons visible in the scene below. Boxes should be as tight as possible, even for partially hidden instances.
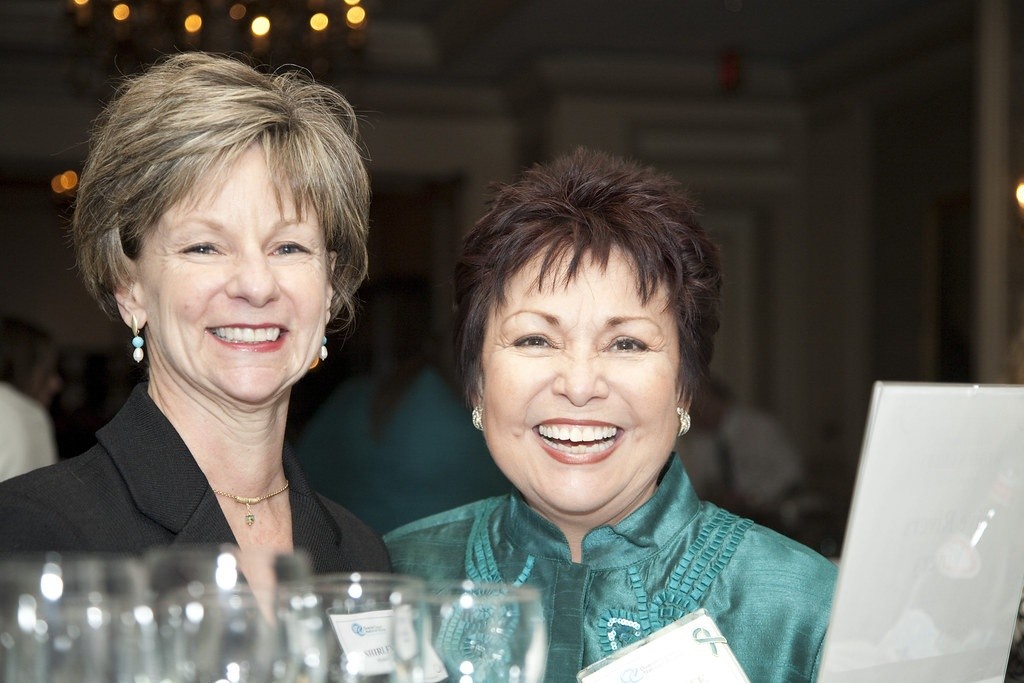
[0,50,396,576]
[382,145,840,683]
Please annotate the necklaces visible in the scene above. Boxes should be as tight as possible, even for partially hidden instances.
[213,479,289,526]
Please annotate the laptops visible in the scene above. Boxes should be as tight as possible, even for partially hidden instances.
[814,381,1024,683]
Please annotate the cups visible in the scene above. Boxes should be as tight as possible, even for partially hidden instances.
[0,540,550,683]
